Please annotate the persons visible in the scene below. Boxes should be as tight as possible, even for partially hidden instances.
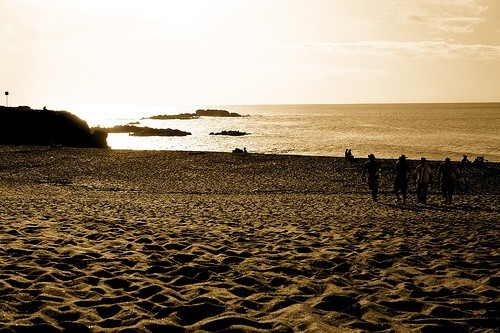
[358,153,382,202]
[412,155,432,204]
[458,154,473,168]
[344,147,356,167]
[435,156,459,204]
[387,153,412,204]
